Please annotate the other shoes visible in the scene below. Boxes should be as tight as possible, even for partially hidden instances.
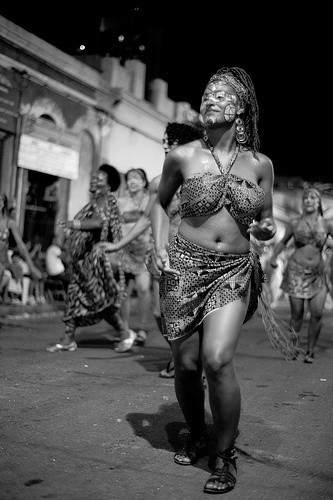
[117,330,137,353]
[136,329,147,345]
[46,341,77,352]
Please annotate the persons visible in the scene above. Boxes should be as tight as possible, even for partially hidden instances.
[46,163,137,354]
[156,66,278,494]
[115,168,155,343]
[0,192,69,306]
[270,188,333,363]
[95,121,208,390]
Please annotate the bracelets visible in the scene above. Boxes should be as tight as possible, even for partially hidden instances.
[73,219,81,230]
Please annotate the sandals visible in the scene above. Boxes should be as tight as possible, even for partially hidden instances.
[174,437,209,465]
[203,448,238,493]
[291,351,298,360]
[160,361,175,378]
[304,350,314,363]
